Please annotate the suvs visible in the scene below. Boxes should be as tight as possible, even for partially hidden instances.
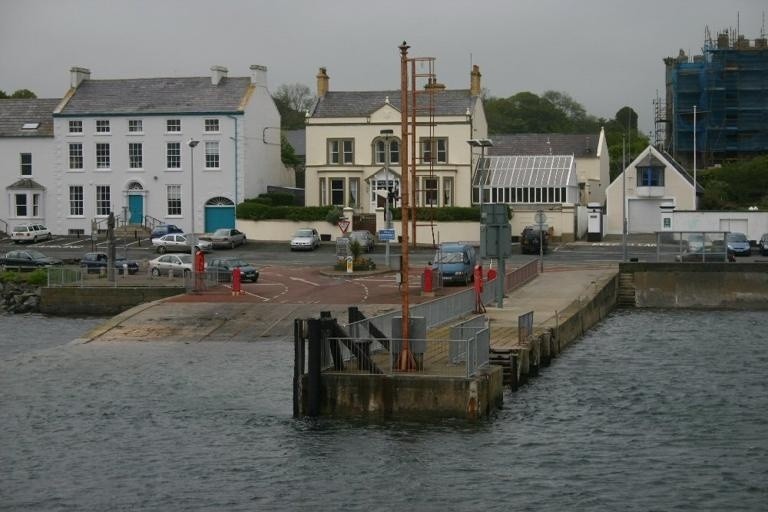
[10,224,52,243]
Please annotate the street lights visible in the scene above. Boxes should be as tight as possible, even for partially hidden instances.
[467,138,494,221]
[186,136,199,247]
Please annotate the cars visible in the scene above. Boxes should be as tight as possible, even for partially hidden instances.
[675,233,767,261]
[146,224,247,255]
[289,229,322,251]
[1,249,64,274]
[78,252,139,276]
[348,229,375,251]
[520,228,549,256]
[146,253,259,284]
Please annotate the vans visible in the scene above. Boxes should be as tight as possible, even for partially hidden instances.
[431,242,477,284]
